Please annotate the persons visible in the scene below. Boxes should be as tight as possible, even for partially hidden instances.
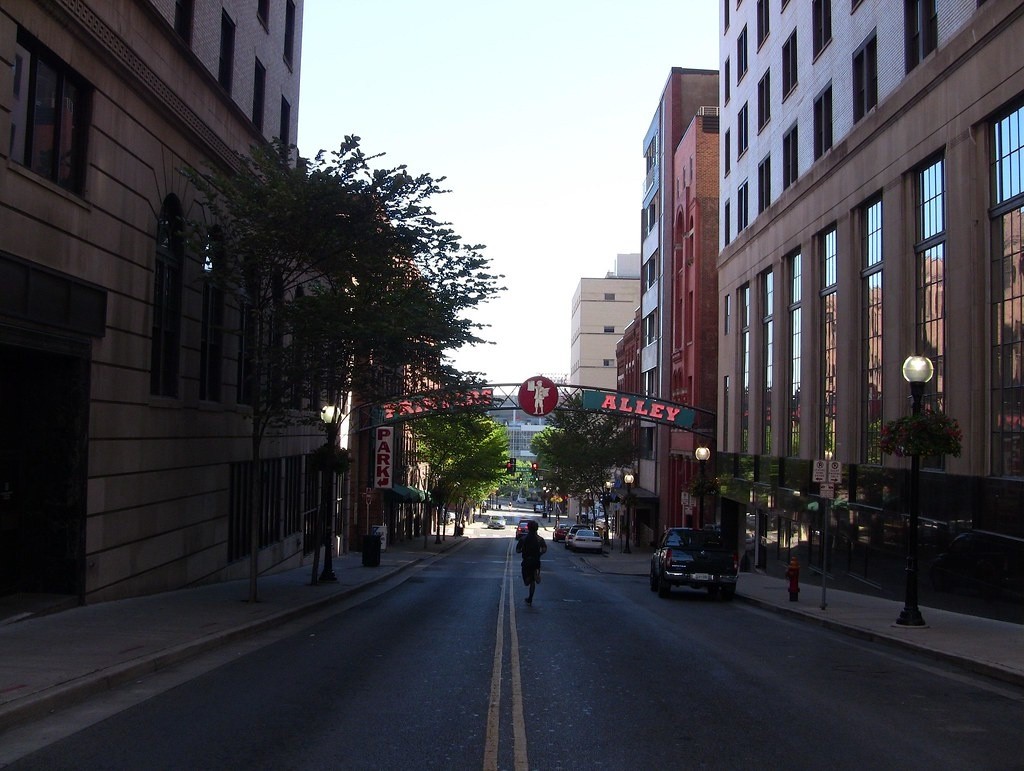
[601,522,610,545]
[516,521,547,606]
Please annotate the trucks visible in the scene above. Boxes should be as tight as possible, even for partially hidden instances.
[588,509,604,522]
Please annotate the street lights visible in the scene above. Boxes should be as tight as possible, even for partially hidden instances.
[695,447,710,529]
[543,487,546,512]
[895,356,934,626]
[624,475,634,554]
[605,482,612,545]
[318,405,341,585]
[586,489,590,525]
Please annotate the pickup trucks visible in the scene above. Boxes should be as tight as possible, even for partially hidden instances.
[649,527,739,601]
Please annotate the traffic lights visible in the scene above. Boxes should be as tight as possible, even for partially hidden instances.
[507,462,511,473]
[532,463,537,474]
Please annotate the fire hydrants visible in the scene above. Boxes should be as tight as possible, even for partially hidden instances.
[786,557,800,601]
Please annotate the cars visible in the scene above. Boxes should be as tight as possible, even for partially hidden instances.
[596,517,615,531]
[488,515,507,529]
[515,519,533,540]
[552,524,590,550]
[571,529,603,553]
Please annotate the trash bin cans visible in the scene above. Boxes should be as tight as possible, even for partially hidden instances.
[361,535,381,567]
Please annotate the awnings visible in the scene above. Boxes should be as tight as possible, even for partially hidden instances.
[391,482,441,507]
[615,485,657,509]
